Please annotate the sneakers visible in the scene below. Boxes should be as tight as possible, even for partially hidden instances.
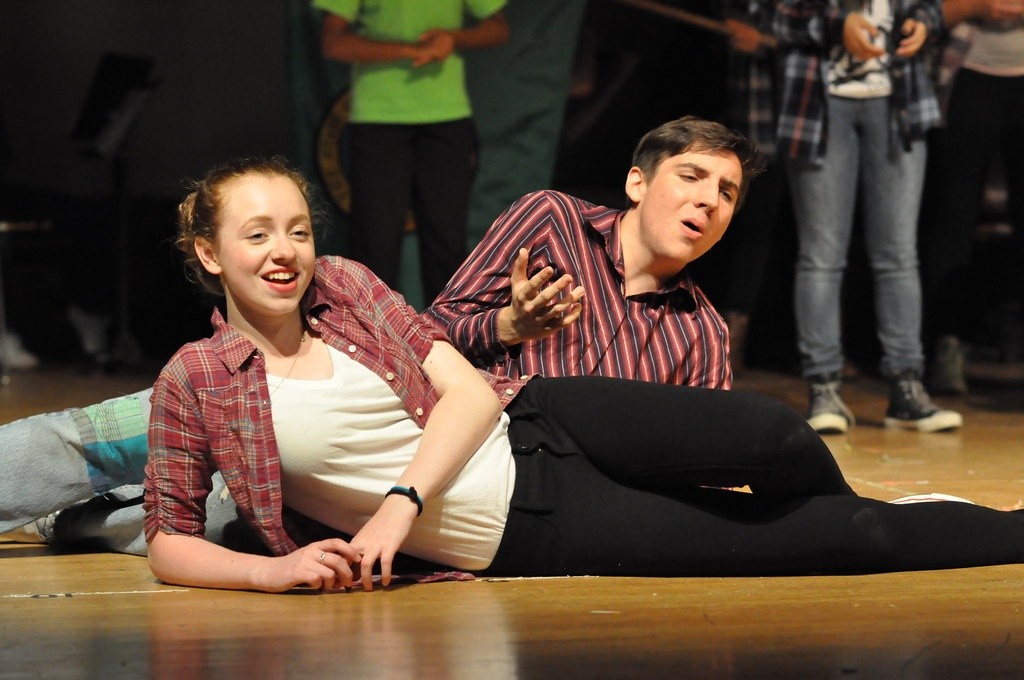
[805,381,855,434]
[22,507,63,544]
[883,375,964,433]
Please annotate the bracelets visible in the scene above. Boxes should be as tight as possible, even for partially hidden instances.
[386,485,425,513]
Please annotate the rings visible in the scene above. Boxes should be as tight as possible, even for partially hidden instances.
[320,552,328,564]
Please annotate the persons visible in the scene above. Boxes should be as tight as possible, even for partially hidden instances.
[933,0,1024,389]
[142,148,1024,595]
[556,24,646,181]
[0,114,754,558]
[768,0,967,433]
[720,0,869,376]
[307,1,515,304]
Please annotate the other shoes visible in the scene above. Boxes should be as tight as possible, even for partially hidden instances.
[938,338,969,392]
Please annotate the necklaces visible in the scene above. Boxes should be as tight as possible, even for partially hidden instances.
[254,331,306,407]
